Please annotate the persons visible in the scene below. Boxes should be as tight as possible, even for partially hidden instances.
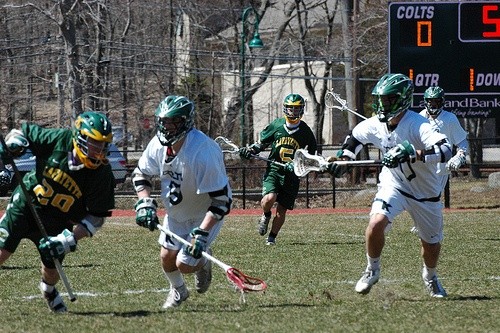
[240,94,318,246]
[412,87,468,231]
[0,112,115,314]
[326,73,453,298]
[130,95,233,309]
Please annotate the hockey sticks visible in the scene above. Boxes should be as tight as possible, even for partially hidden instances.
[0,133,78,302]
[154,221,266,292]
[213,136,287,168]
[293,149,415,177]
[324,92,369,120]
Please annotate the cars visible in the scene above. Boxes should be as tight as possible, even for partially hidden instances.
[0,142,129,193]
[112,125,133,145]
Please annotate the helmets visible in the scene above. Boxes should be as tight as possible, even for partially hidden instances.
[370,73,414,122]
[423,87,445,115]
[71,111,112,170]
[282,94,305,124]
[154,95,195,145]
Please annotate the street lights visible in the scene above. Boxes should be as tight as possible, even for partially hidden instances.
[240,7,264,166]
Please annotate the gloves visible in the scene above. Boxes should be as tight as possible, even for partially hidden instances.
[239,144,259,160]
[327,151,356,177]
[445,153,466,172]
[38,228,76,261]
[0,134,29,164]
[284,162,295,172]
[184,228,209,259]
[382,139,417,168]
[134,197,161,231]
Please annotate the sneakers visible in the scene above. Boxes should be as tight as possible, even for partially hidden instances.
[266,241,274,247]
[38,281,67,313]
[194,248,212,294]
[354,266,380,294]
[161,284,190,310]
[423,275,447,298]
[258,212,272,236]
